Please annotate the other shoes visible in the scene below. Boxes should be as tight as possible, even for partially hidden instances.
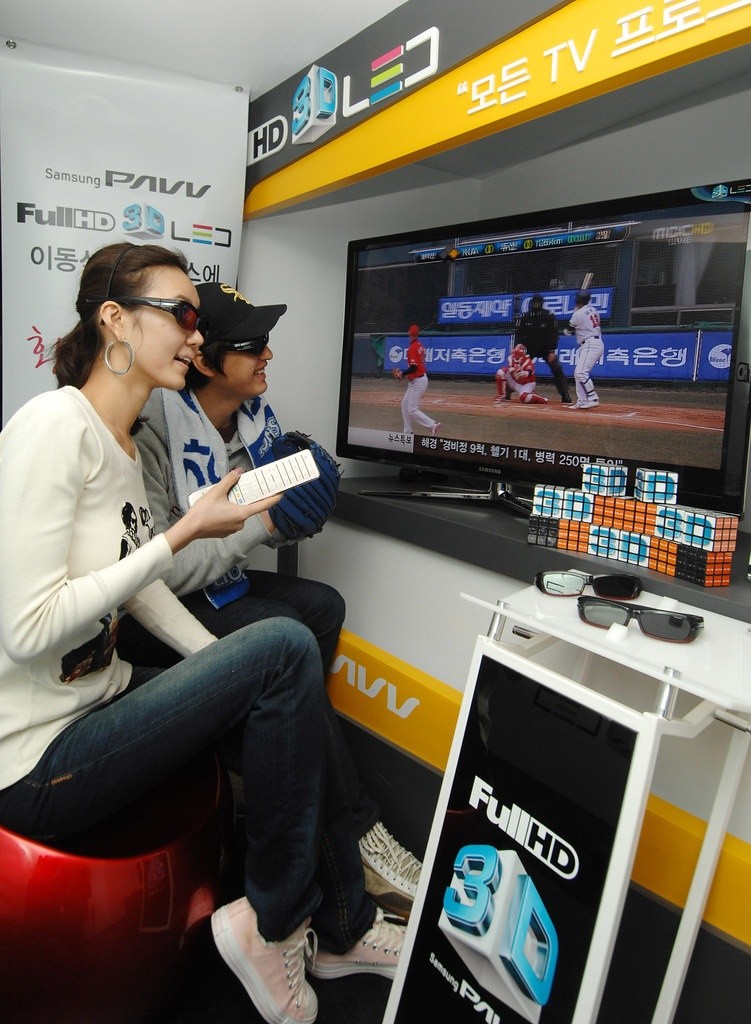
[581,402,599,409]
[561,392,572,403]
[570,403,580,409]
[494,395,506,401]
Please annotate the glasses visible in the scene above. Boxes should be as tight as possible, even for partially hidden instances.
[578,596,703,644]
[218,332,269,356]
[534,570,642,602]
[99,296,210,343]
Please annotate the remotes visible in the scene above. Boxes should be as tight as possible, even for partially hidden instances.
[187,449,320,511]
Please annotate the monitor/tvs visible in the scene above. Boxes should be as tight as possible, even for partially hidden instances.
[336,179,751,519]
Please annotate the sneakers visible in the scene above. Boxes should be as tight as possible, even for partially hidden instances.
[352,821,422,911]
[211,896,319,1024]
[432,422,441,436]
[304,907,409,981]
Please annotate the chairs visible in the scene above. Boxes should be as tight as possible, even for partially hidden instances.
[0,748,233,1024]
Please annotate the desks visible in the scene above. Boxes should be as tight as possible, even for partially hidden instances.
[280,475,751,955]
[383,569,751,1024]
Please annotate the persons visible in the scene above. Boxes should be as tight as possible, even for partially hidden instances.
[0,241,423,1024]
[490,290,603,409]
[392,325,441,435]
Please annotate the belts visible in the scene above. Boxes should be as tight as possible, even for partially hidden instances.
[411,375,425,380]
[577,336,599,348]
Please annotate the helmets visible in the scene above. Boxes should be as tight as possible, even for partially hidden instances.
[513,344,527,356]
[576,291,590,304]
[528,296,543,311]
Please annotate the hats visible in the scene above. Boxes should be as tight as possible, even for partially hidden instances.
[187,282,287,354]
[409,325,419,336]
